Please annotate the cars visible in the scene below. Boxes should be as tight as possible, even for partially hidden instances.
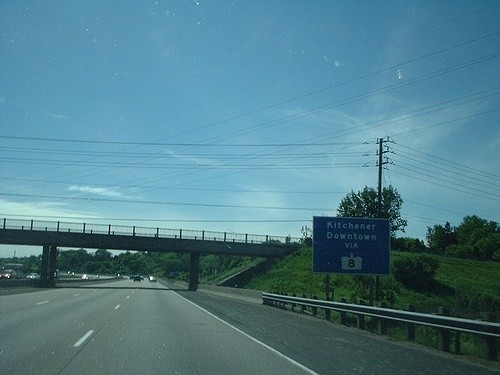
[54,267,178,284]
[0,270,17,279]
[25,272,40,279]
